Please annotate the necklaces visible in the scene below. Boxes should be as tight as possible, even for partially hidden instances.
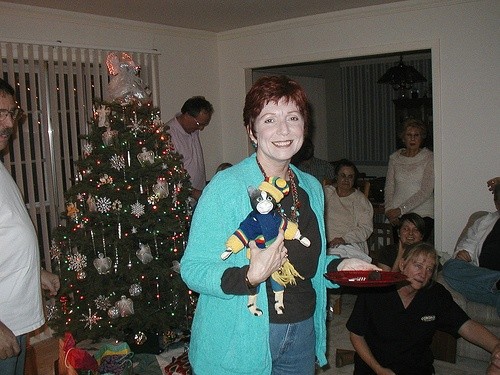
[255,155,300,223]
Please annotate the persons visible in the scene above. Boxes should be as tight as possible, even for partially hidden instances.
[391,212,426,272]
[345,241,500,375]
[384,118,435,258]
[442,177,500,314]
[164,97,215,213]
[292,137,335,185]
[179,74,382,375]
[0,78,60,375]
[321,160,373,320]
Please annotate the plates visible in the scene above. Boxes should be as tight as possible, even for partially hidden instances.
[324,271,408,287]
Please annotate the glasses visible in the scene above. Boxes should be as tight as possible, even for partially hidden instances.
[190,116,204,130]
[0,108,24,122]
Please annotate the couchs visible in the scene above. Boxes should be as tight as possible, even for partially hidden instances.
[436,211,500,375]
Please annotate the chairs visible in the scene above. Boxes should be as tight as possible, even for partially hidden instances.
[324,179,371,199]
[366,222,394,266]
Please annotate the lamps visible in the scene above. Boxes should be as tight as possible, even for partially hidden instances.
[376,53,427,98]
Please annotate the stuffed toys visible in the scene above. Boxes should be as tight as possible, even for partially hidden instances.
[221,177,310,316]
